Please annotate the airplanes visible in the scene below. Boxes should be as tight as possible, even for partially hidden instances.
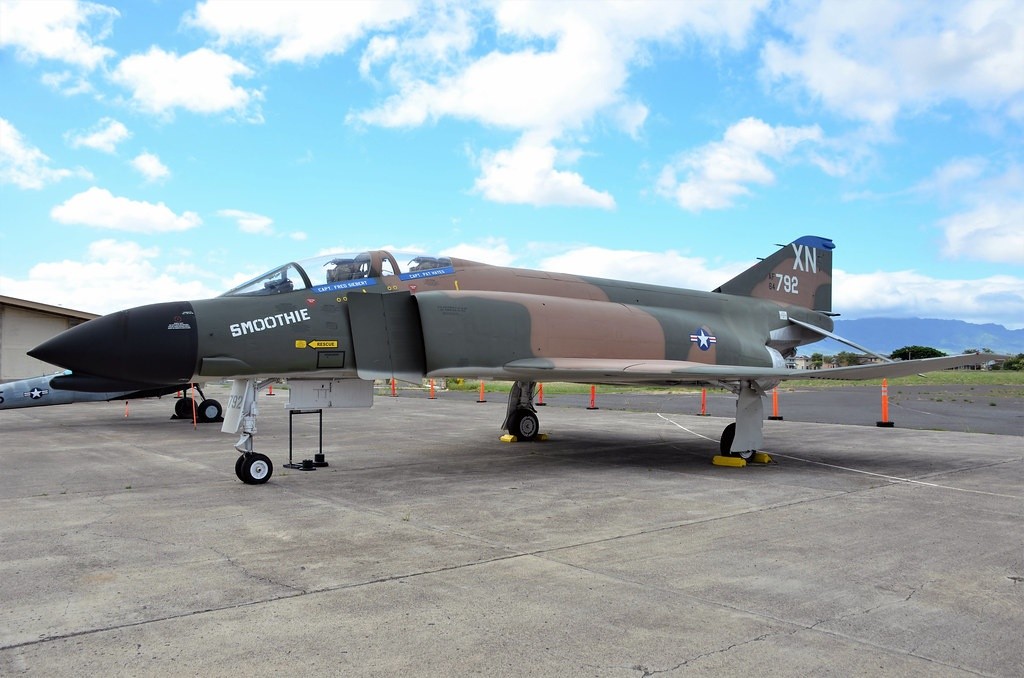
[1,370,223,423]
[27,236,1013,486]
